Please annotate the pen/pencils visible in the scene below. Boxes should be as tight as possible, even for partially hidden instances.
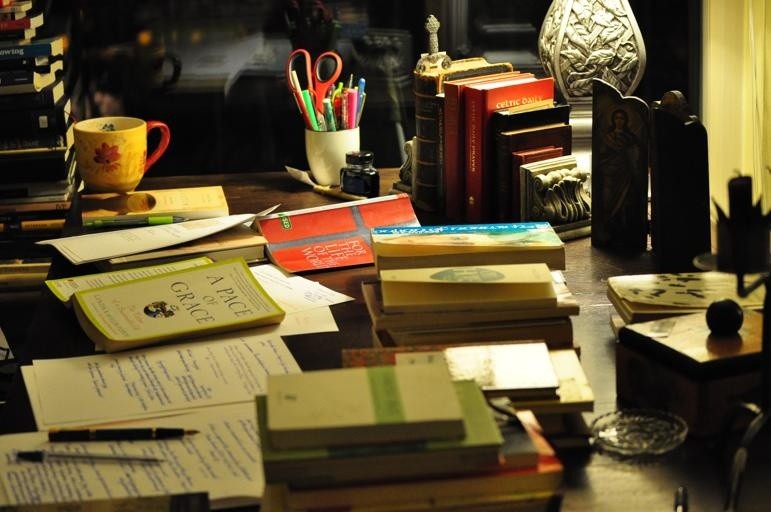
[16,451,170,464]
[47,428,200,442]
[676,485,686,511]
[9,216,186,232]
[315,185,368,201]
[291,70,367,132]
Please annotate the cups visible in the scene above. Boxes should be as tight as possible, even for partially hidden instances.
[73,117,171,193]
[102,45,183,110]
[305,126,361,186]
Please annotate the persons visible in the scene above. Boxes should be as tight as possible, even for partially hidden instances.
[595,108,646,254]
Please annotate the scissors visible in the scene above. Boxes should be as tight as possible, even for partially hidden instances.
[286,50,342,130]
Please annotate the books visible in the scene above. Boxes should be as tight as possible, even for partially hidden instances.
[605,268,766,343]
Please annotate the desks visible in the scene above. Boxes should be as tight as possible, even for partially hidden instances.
[0,167,771,512]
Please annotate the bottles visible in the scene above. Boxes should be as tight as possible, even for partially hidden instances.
[340,151,380,198]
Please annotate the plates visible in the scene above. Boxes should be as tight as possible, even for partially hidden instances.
[590,408,690,457]
